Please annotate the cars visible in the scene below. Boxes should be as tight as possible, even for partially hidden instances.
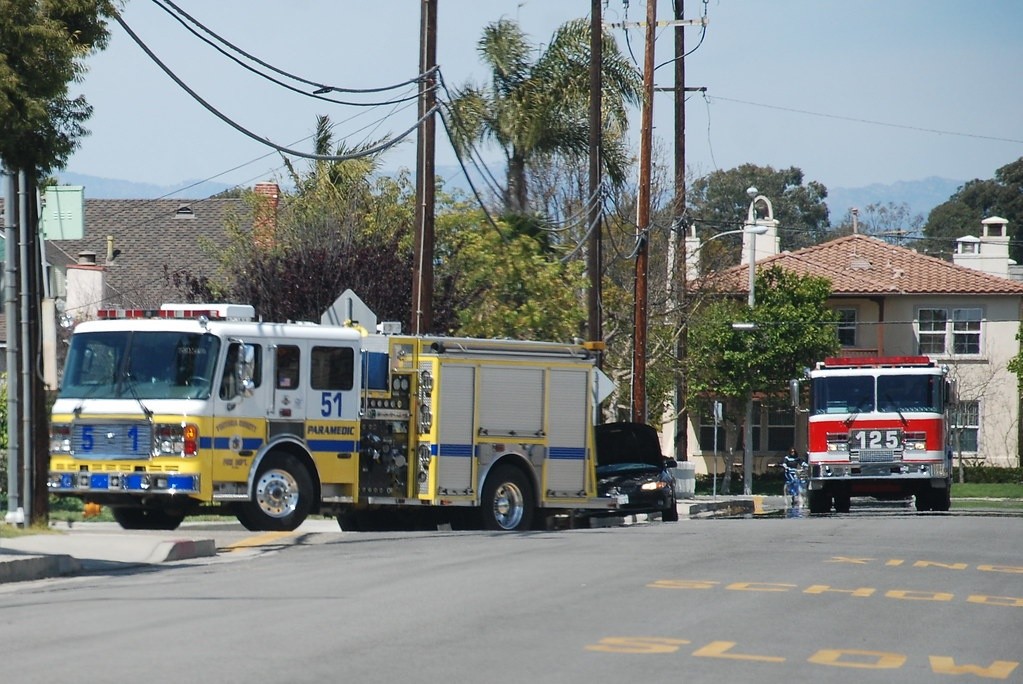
[570,422,679,526]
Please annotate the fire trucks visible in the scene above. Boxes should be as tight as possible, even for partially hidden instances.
[790,355,958,512]
[44,303,596,535]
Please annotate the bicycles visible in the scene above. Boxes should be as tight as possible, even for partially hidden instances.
[784,467,806,509]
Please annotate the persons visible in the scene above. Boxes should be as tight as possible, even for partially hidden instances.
[176,346,233,398]
[895,380,921,408]
[783,448,808,496]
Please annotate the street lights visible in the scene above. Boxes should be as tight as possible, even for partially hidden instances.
[673,227,768,457]
